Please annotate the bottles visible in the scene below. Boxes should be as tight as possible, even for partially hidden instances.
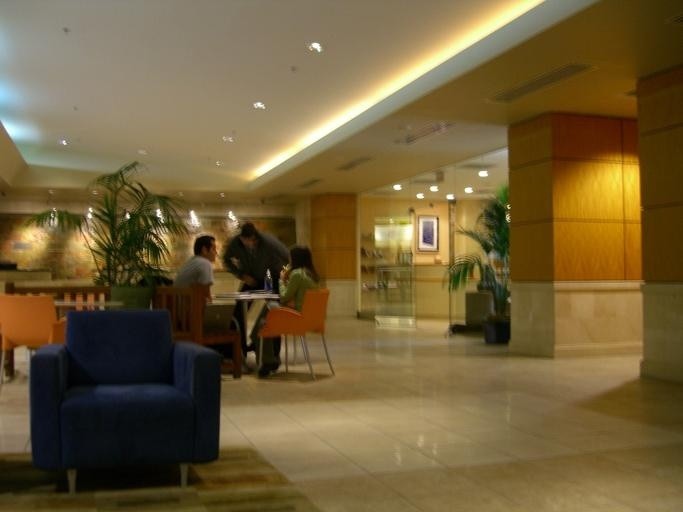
[264,269,273,294]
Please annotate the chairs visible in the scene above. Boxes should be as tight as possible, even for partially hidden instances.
[29,310,222,493]
[0,283,335,380]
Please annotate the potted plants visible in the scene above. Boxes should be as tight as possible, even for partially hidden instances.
[441,176,510,345]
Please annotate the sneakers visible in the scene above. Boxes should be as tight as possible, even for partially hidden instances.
[258,361,282,378]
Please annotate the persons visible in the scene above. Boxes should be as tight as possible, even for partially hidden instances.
[254,246,320,376]
[220,222,289,366]
[177,232,251,374]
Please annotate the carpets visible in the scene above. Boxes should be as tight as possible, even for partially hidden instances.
[0,443,320,512]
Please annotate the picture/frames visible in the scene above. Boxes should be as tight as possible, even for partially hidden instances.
[417,215,439,254]
